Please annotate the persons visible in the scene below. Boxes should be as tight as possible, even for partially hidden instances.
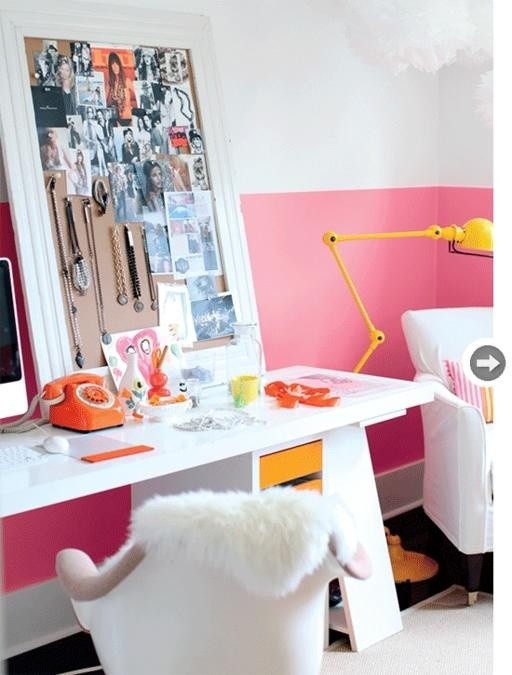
[137,156,165,216]
[31,39,211,196]
[111,162,129,220]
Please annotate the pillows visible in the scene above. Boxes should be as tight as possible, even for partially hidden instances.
[442,358,492,424]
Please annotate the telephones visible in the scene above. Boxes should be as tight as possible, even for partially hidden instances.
[43,373,125,434]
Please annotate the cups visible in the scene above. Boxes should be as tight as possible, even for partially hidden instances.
[231,376,259,405]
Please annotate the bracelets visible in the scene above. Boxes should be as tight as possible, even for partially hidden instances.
[91,178,110,214]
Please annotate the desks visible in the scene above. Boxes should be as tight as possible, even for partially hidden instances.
[0,368,435,675]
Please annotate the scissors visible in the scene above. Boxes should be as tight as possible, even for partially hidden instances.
[152,349,162,368]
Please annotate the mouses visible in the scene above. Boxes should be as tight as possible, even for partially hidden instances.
[42,434,70,454]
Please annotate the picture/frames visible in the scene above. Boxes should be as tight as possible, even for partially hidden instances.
[0,0,267,424]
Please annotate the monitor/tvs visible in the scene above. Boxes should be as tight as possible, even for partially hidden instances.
[0,256,31,421]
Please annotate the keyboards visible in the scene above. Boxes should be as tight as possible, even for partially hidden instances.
[0,443,44,476]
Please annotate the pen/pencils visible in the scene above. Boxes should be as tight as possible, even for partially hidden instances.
[160,345,170,368]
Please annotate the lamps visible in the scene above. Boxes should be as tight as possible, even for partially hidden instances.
[322,217,493,374]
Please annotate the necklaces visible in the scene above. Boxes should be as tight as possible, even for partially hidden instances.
[109,224,130,306]
[80,196,113,346]
[63,196,91,296]
[141,228,158,312]
[47,174,84,369]
[123,224,145,313]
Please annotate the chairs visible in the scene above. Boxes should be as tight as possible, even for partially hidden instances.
[55,491,371,674]
[401,307,492,605]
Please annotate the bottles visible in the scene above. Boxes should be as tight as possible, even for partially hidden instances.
[224,321,262,393]
[187,378,201,405]
